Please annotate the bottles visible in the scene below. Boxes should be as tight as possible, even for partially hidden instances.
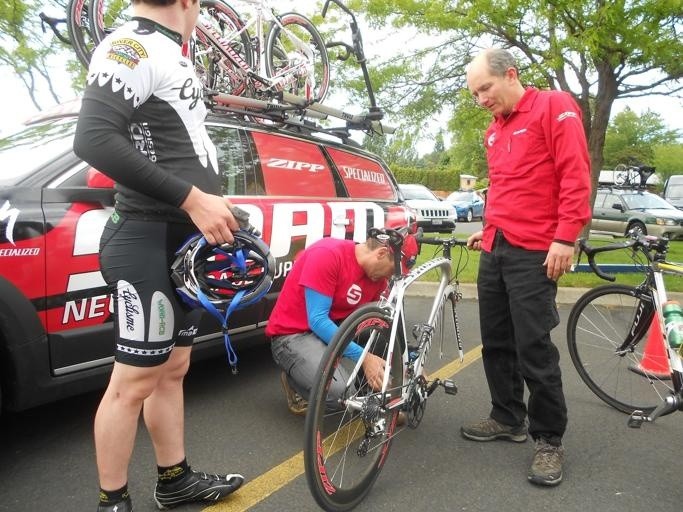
[661,299,682,347]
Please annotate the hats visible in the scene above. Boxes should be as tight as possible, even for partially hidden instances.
[387,226,419,276]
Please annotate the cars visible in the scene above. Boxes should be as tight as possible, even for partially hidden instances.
[440,189,485,222]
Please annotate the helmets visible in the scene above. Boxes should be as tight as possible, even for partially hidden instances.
[171,230,275,312]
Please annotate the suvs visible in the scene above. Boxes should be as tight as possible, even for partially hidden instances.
[396,183,457,234]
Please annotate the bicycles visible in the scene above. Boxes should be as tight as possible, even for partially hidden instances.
[566,215,682,428]
[41,0,397,144]
[303,225,484,511]
[612,164,644,188]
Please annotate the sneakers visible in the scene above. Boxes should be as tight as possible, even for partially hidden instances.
[281,371,310,415]
[527,438,564,485]
[461,416,528,442]
[153,465,245,510]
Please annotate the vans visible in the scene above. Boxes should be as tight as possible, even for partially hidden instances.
[0,106,416,416]
[663,174,682,212]
[588,190,682,241]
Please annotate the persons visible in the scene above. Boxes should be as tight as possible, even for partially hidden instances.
[71,0,247,512]
[458,48,594,486]
[259,221,418,428]
